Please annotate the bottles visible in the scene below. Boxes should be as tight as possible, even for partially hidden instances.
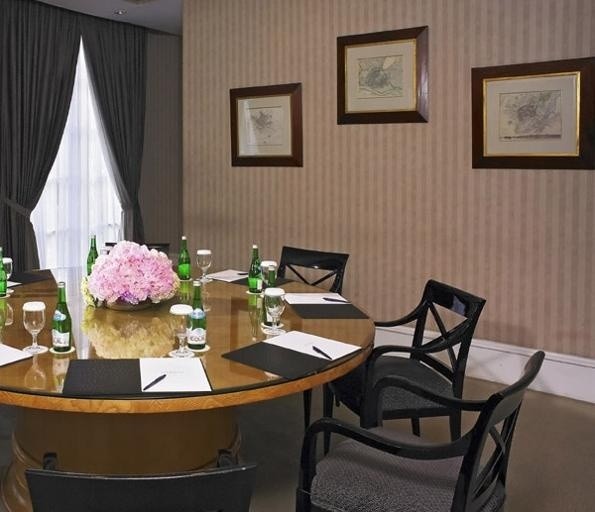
[85,234,99,274]
[51,281,71,350]
[187,280,207,347]
[0,298,6,329]
[176,235,191,280]
[0,249,8,297]
[191,350,207,373]
[176,280,189,303]
[51,353,69,389]
[246,244,287,344]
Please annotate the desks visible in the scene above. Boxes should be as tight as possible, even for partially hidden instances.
[1,271,375,512]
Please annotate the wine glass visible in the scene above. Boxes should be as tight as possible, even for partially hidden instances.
[3,258,15,293]
[19,300,48,353]
[195,250,214,281]
[197,280,213,311]
[2,292,15,326]
[168,304,196,358]
[24,353,47,390]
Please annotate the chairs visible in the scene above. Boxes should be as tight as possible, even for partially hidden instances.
[277,246,349,431]
[320,279,486,454]
[294,351,545,512]
[105,241,170,281]
[24,449,258,512]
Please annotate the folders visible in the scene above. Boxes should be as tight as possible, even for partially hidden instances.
[62,358,215,396]
[5,270,50,287]
[213,272,294,290]
[284,299,370,319]
[221,341,363,381]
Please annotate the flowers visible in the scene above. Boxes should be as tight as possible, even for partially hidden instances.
[86,241,180,305]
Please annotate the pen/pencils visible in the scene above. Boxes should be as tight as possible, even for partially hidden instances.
[322,297,347,302]
[312,346,332,360]
[143,374,166,390]
[237,273,249,275]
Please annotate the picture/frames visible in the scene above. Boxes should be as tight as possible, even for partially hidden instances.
[472,56,595,169]
[230,82,303,166]
[336,25,429,124]
